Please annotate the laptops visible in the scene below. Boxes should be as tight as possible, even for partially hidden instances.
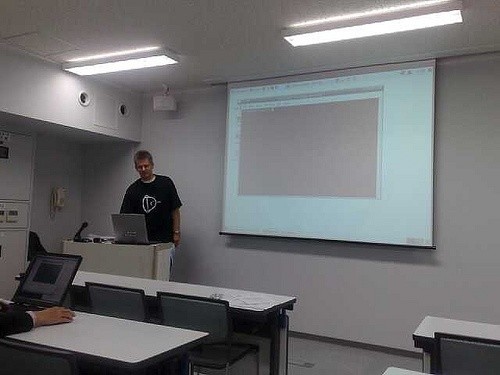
[111,213,164,244]
[6,252,84,312]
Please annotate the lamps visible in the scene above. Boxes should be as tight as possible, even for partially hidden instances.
[281,0,464,48]
[62,47,181,77]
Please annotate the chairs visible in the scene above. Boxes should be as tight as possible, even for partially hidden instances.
[0,337,80,375]
[156,291,260,375]
[434,332,500,375]
[85,282,147,323]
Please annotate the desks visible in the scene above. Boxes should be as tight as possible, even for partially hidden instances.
[6,311,209,375]
[71,270,297,375]
[382,315,500,375]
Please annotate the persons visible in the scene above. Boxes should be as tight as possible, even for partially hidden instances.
[0,306,75,337]
[120,149,182,271]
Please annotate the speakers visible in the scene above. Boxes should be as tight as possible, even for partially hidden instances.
[153,95,178,112]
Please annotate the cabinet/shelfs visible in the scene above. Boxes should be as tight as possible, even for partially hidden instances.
[0,131,38,300]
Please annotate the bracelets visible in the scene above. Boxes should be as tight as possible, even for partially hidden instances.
[173,231,181,234]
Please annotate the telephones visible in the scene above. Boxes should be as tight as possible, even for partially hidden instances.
[53,188,65,208]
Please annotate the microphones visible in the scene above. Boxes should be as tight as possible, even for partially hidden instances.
[74,222,88,241]
